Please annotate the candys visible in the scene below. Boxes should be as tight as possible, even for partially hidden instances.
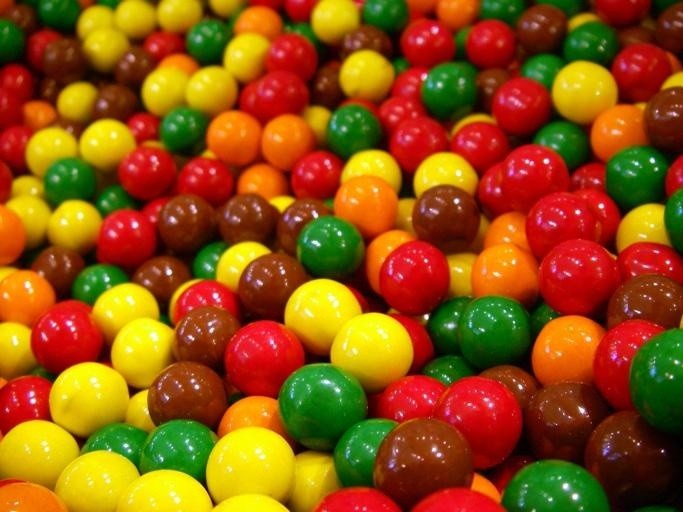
[0,0,683,512]
